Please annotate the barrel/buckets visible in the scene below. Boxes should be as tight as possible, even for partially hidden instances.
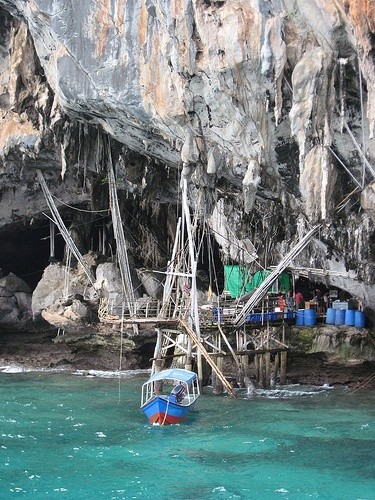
[345,310,355,326]
[295,309,305,326]
[304,309,316,326]
[326,308,336,325]
[334,309,345,326]
[355,310,365,328]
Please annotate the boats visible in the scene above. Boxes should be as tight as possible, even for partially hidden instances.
[141,367,202,428]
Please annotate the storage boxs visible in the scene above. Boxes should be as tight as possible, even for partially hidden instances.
[247,307,293,321]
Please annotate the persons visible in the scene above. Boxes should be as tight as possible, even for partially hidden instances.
[286,288,328,316]
